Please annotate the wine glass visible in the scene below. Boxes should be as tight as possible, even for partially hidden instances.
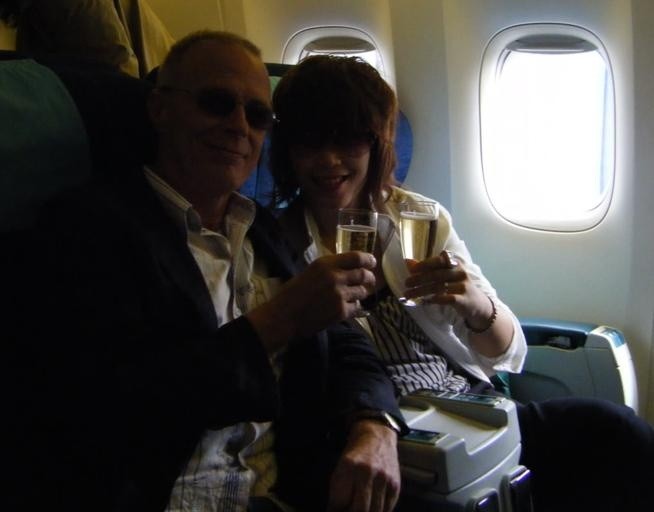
[398,201,440,307]
[334,207,378,318]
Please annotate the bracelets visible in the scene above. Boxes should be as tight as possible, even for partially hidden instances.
[463,295,497,335]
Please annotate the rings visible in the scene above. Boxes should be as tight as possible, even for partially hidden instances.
[440,251,450,267]
[442,280,448,296]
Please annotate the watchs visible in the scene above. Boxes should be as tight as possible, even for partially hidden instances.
[349,408,403,440]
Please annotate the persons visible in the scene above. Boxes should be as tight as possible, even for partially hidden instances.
[261,53,652,512]
[0,29,413,512]
[0,0,164,160]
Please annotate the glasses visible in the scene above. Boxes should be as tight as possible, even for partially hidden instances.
[289,129,376,159]
[157,83,281,131]
